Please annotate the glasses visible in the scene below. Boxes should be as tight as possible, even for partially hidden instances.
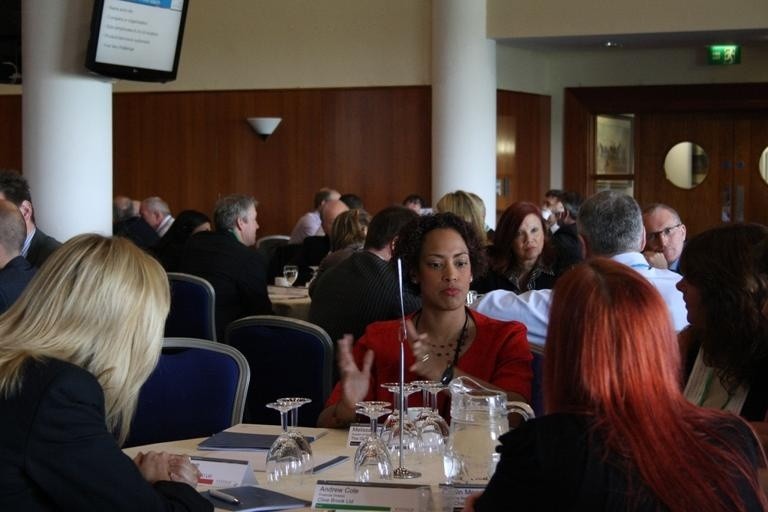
[647,225,681,240]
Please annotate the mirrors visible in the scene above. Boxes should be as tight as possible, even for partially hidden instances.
[663,140,711,191]
[758,146,768,185]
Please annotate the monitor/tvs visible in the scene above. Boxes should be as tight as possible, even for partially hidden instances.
[86,0,190,82]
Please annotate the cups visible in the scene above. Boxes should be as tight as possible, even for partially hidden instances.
[448,373,535,483]
[275,277,289,287]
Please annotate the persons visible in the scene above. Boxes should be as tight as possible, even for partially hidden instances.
[0,232,218,512]
[318,210,535,431]
[1,170,65,266]
[291,189,493,356]
[675,221,764,510]
[0,200,36,313]
[198,484,312,510]
[460,255,767,509]
[480,189,690,352]
[113,194,273,341]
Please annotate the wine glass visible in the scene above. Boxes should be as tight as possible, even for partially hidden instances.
[354,380,444,483]
[277,397,314,482]
[266,402,305,493]
[284,265,299,287]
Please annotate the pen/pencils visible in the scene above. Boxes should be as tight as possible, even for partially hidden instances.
[208,489,240,504]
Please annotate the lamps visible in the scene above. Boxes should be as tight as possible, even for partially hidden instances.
[246,116,283,143]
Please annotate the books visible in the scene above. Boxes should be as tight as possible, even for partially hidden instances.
[198,431,316,453]
